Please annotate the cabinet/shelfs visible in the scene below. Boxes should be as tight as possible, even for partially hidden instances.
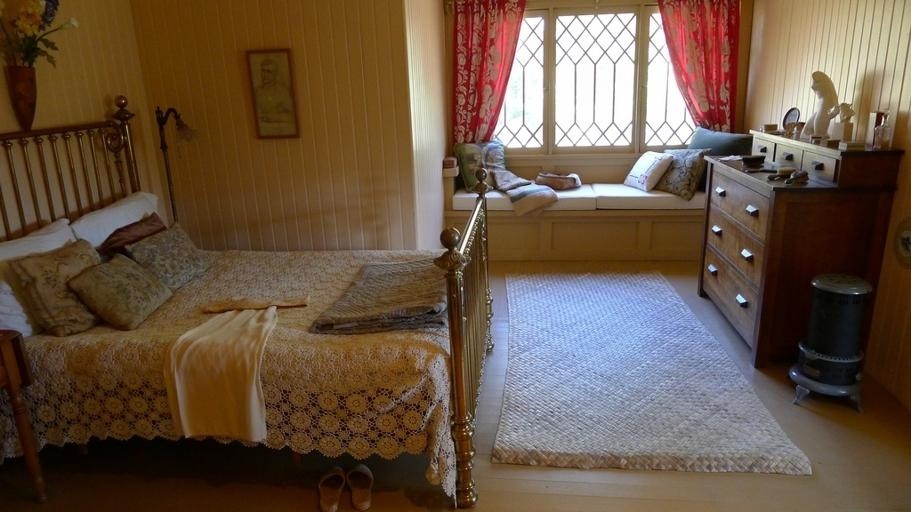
[694,129,902,371]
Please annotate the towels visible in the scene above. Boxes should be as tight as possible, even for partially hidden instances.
[163,307,277,445]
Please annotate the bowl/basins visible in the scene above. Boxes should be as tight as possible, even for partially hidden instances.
[763,124,777,133]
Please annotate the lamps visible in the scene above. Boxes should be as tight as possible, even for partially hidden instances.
[154,102,204,223]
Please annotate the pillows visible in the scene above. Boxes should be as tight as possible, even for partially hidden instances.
[59,252,176,329]
[621,148,673,193]
[3,238,103,335]
[124,217,212,289]
[688,125,754,193]
[104,211,167,253]
[0,218,80,338]
[454,140,509,194]
[64,190,161,247]
[660,145,710,200]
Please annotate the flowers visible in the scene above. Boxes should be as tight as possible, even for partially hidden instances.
[1,1,78,68]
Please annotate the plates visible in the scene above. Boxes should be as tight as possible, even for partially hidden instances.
[783,107,800,129]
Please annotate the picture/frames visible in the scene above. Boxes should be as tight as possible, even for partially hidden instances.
[244,47,302,139]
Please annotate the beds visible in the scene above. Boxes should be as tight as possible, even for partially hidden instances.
[0,97,493,511]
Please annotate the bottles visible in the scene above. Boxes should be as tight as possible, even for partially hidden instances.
[873,114,894,150]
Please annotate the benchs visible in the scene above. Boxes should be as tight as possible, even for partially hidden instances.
[441,152,706,263]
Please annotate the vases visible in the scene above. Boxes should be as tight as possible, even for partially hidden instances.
[4,66,38,133]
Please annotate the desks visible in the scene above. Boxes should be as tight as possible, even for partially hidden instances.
[0,328,49,502]
